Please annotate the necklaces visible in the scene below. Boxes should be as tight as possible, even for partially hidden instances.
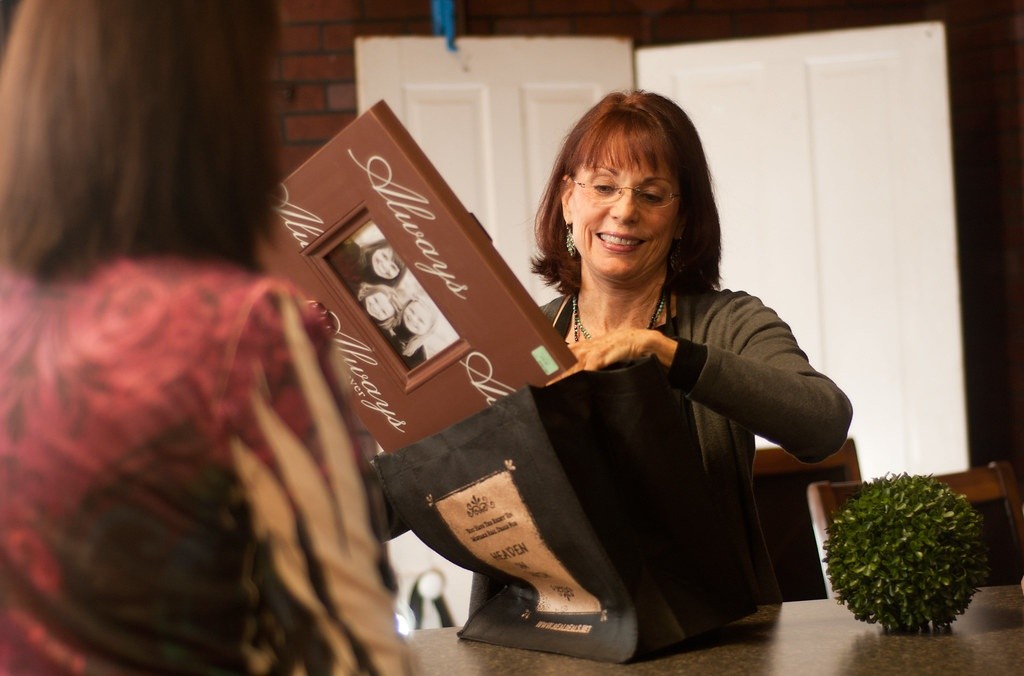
[572,293,665,343]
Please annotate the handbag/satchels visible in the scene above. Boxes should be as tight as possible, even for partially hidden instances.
[364,353,760,666]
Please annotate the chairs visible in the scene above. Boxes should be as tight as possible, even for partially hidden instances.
[753,439,862,601]
[806,460,1024,600]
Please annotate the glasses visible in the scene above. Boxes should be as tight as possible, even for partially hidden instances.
[563,178,695,208]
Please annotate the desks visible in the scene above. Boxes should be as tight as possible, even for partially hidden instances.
[404,586,1024,676]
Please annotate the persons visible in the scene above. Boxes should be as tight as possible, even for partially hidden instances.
[324,236,435,371]
[0,0,421,676]
[523,90,854,608]
